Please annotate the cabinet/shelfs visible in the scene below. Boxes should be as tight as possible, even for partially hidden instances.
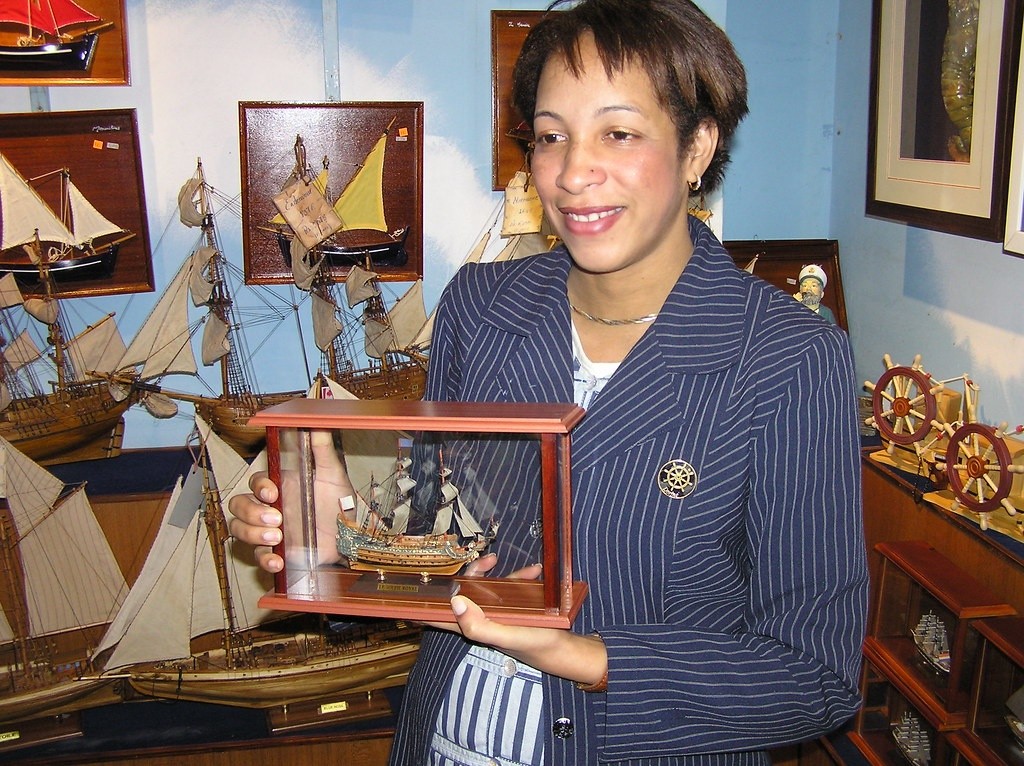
[859,434,1024,717]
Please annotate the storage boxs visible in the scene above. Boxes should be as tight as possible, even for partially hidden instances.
[846,541,1024,766]
[246,399,588,631]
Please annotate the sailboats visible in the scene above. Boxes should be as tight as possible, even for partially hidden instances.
[891,712,933,766]
[335,436,501,576]
[910,609,951,676]
[387,144,563,377]
[83,157,431,461]
[0,435,143,727]
[0,151,145,467]
[0,0,116,76]
[0,166,137,285]
[259,114,411,261]
[66,370,422,711]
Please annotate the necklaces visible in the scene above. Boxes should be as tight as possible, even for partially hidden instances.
[568,303,660,325]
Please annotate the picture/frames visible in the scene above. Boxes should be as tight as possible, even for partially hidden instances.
[238,100,424,286]
[0,0,132,87]
[0,109,156,302]
[491,10,563,190]
[865,0,1024,260]
[721,238,848,338]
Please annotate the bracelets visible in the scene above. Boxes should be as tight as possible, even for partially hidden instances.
[577,633,609,692]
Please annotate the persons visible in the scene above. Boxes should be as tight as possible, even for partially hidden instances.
[790,264,837,326]
[229,0,870,766]
